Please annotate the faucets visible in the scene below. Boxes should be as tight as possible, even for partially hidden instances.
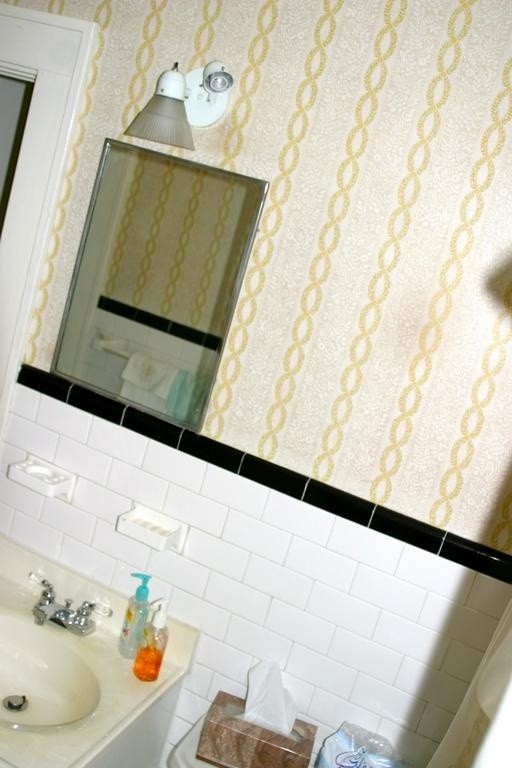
[32,603,71,625]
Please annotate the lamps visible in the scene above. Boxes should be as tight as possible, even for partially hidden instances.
[124,60,234,151]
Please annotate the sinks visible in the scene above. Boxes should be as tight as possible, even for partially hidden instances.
[0,610,101,730]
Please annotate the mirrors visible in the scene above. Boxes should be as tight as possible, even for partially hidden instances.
[49,137,270,438]
